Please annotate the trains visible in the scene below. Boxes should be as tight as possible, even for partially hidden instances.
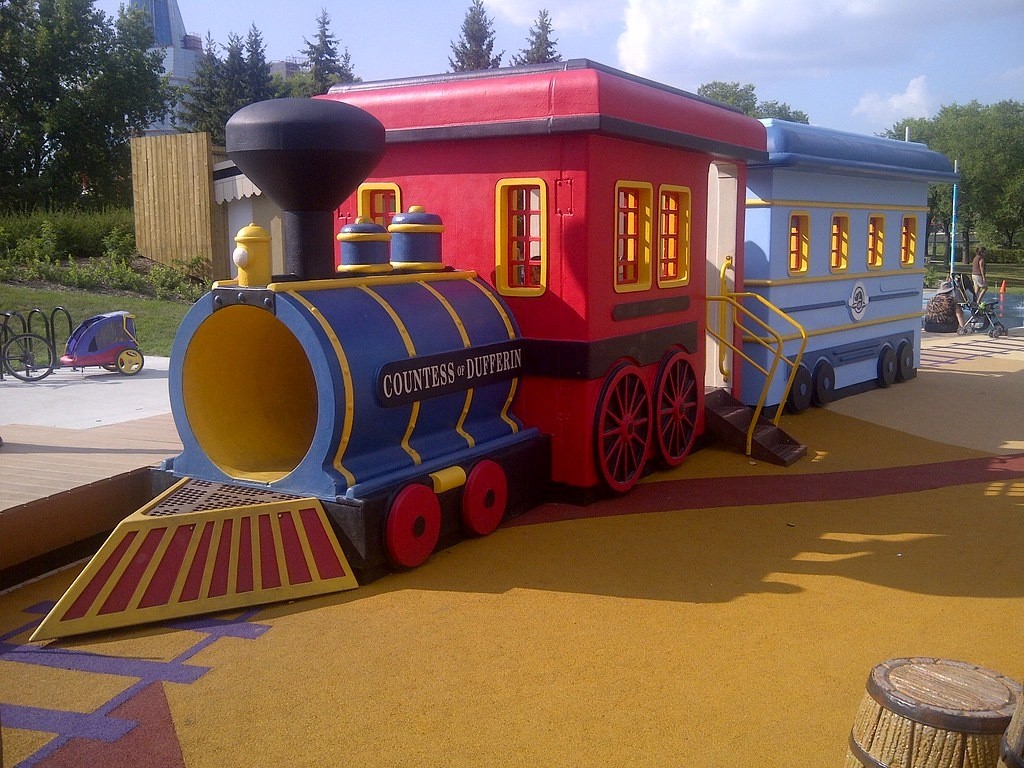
[161,58,963,571]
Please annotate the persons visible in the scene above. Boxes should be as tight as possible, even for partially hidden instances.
[971,247,987,306]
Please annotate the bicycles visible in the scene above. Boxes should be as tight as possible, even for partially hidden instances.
[0,311,59,382]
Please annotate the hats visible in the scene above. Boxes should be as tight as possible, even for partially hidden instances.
[937,282,954,293]
[979,247,989,252]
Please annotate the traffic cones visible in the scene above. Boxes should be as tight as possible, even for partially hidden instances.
[999,280,1007,293]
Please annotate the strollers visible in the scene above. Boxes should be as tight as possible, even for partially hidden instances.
[951,272,1009,339]
